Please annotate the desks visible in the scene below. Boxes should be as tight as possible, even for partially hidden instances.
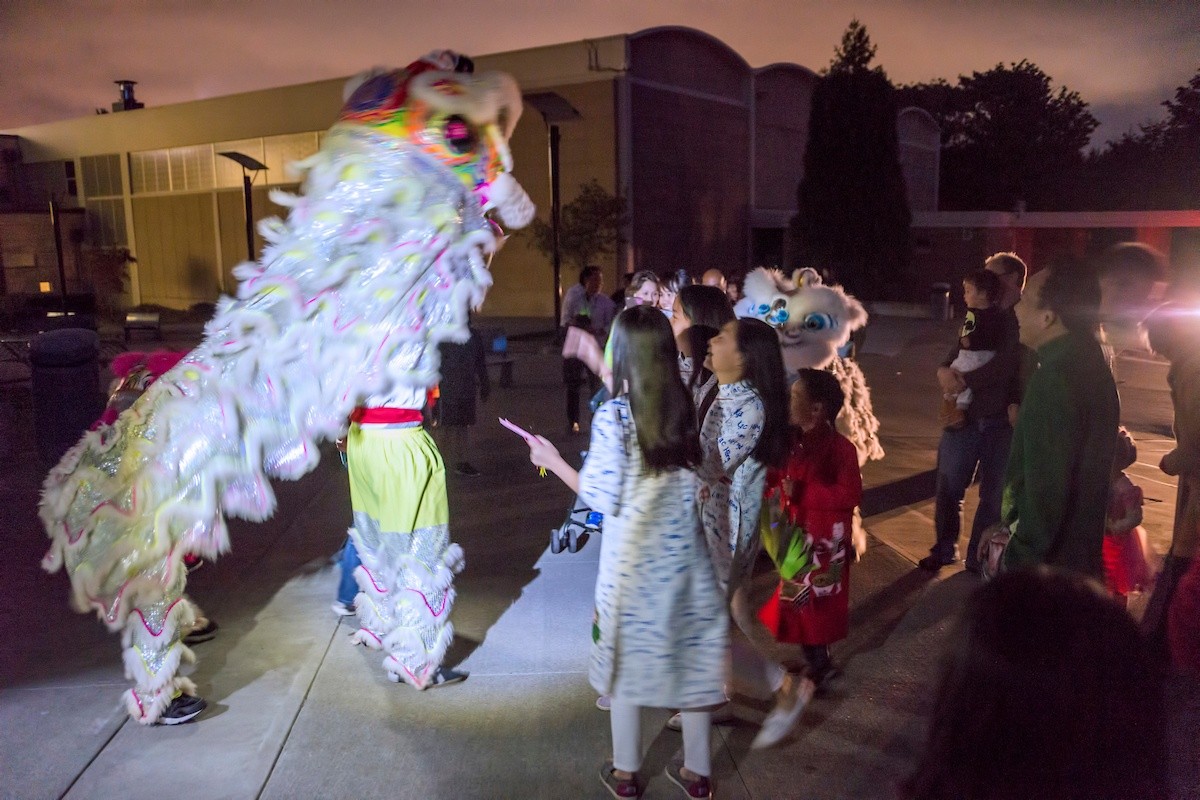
[1,329,41,366]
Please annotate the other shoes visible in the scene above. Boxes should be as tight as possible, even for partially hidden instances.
[596,695,611,710]
[663,763,711,800]
[666,700,733,730]
[599,762,637,800]
[754,676,816,749]
[558,420,579,439]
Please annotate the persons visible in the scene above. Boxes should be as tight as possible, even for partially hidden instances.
[900,244,1200,800]
[526,306,733,800]
[561,263,863,749]
[421,308,491,478]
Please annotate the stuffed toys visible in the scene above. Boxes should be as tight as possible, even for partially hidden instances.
[733,267,885,564]
[41,50,537,725]
[90,350,203,574]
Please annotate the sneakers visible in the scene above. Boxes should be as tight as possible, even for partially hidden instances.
[157,693,206,725]
[430,667,468,686]
[919,553,953,573]
[939,402,955,418]
[333,600,357,617]
[944,408,965,430]
[182,621,217,642]
[456,463,481,477]
[183,554,203,573]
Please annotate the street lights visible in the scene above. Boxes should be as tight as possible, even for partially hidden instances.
[525,92,583,342]
[218,152,269,261]
[29,164,69,319]
